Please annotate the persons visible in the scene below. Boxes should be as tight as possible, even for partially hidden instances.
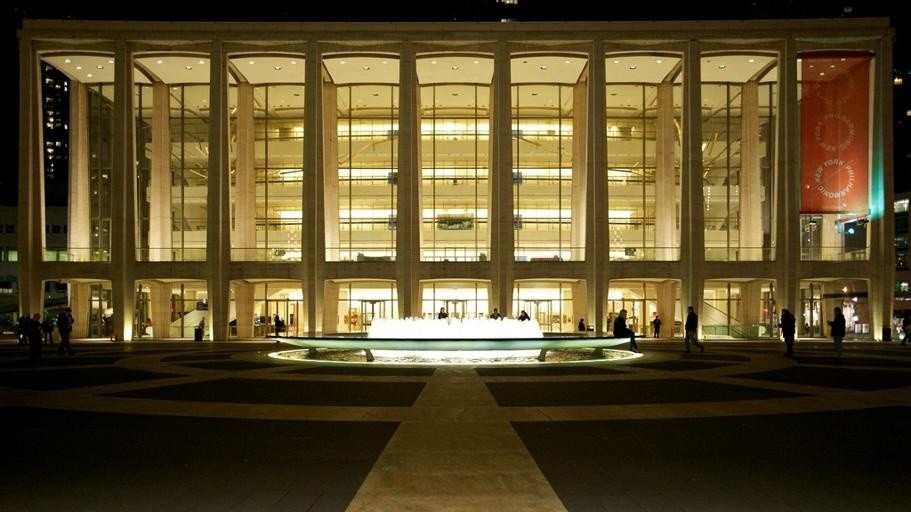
[653,315,662,337]
[519,311,530,320]
[490,307,501,320]
[683,305,704,353]
[826,307,846,356]
[612,310,643,354]
[14,304,76,358]
[607,313,612,331]
[898,312,910,344]
[437,307,447,320]
[578,318,585,331]
[200,315,205,337]
[778,309,796,358]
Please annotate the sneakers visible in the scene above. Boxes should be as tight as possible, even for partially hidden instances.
[700,345,704,352]
[683,351,691,353]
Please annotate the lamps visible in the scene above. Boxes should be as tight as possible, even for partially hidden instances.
[809,221,816,224]
[856,219,869,226]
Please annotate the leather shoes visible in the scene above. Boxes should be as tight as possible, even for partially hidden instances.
[630,350,642,353]
